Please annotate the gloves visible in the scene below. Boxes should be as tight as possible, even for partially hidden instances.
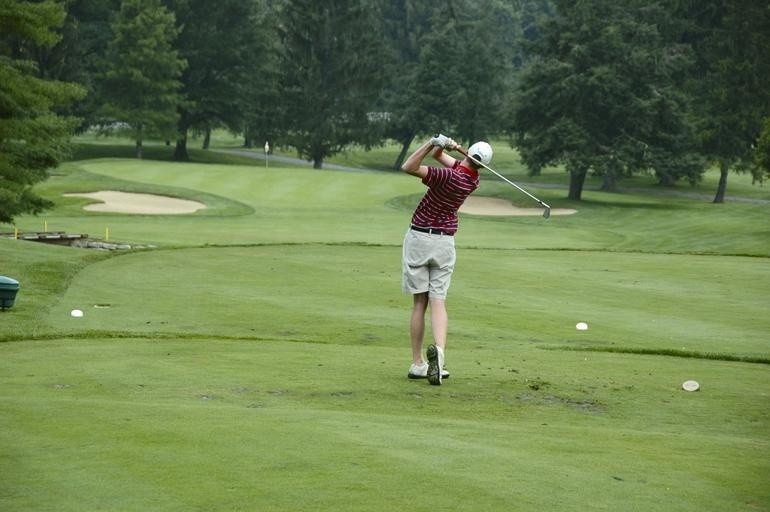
[445,138,461,153]
[430,136,452,148]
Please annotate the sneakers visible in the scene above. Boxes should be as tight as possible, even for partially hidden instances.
[426,344,444,385]
[407,362,449,379]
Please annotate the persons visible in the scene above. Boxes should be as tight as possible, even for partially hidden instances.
[401,134,493,386]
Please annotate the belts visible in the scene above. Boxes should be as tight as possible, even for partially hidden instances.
[410,225,450,236]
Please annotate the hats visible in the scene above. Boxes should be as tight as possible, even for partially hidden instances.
[467,141,493,168]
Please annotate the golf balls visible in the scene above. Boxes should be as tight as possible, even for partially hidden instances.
[576,321,587,332]
[70,308,83,317]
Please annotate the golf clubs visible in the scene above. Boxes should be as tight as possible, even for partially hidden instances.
[435,134,550,218]
